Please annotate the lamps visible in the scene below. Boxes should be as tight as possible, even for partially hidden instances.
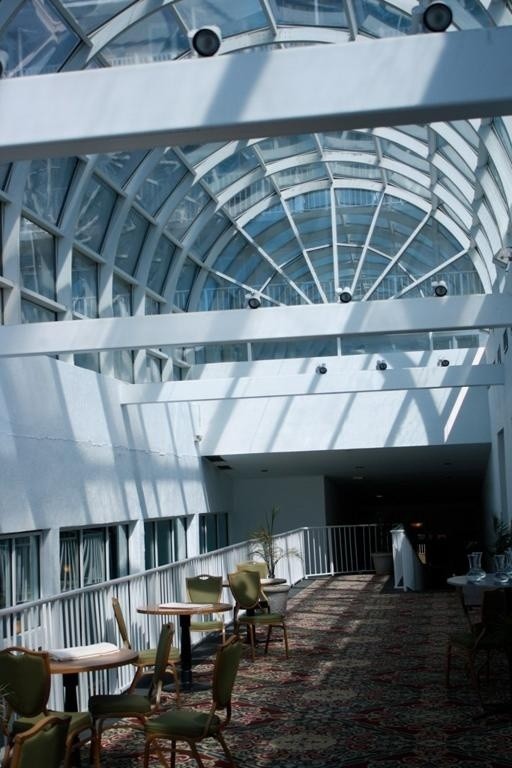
[246,279,447,309]
[345,279,347,281]
[317,356,451,373]
[189,2,454,57]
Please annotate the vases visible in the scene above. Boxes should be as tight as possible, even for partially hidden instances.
[371,553,393,576]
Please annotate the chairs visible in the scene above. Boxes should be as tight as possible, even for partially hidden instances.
[185,574,226,644]
[144,642,241,768]
[446,568,512,688]
[227,570,288,663]
[111,598,182,702]
[89,620,174,768]
[236,563,271,613]
[1,645,94,767]
[2,713,71,767]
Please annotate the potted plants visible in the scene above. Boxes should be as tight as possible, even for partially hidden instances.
[246,504,304,620]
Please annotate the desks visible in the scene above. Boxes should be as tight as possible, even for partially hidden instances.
[46,648,137,713]
[139,602,233,694]
[221,578,288,642]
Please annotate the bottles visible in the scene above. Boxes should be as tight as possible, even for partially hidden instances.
[466,552,510,584]
